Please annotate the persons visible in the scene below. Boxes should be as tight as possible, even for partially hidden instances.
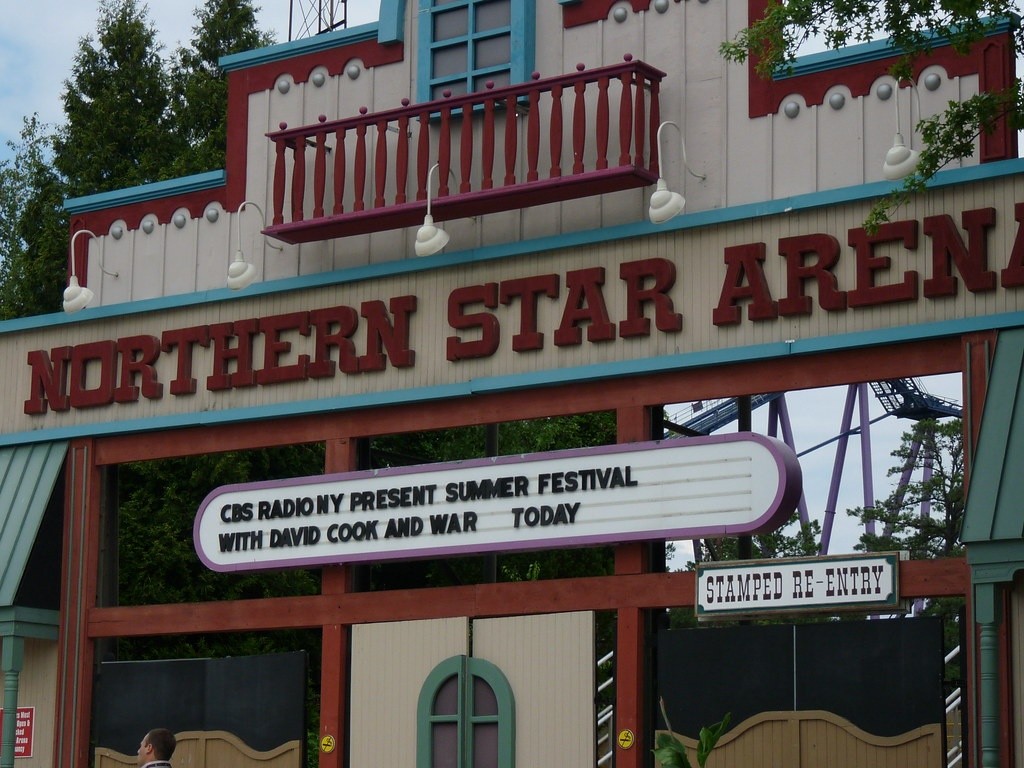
[136,729,176,768]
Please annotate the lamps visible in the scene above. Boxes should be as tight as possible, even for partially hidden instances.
[649,120,704,225]
[63,229,118,312]
[415,163,457,257]
[884,76,927,179]
[226,202,282,292]
